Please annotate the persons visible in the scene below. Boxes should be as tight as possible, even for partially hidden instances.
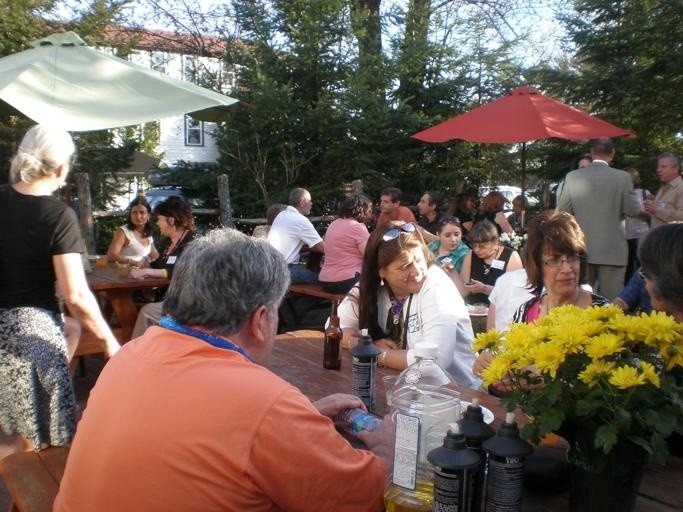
[0,124,120,453]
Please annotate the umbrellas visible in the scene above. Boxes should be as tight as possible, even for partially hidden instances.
[0,23,240,205]
[409,85,637,253]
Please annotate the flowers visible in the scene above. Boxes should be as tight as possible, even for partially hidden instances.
[471,301,682,482]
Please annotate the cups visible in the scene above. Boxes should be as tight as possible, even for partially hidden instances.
[94,257,130,278]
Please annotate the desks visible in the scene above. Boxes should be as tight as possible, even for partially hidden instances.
[254,328,681,512]
[55,258,169,380]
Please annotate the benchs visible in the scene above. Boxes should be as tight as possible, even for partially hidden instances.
[2,446,73,512]
[285,282,349,326]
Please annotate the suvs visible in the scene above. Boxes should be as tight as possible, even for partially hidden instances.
[479,185,538,219]
[119,181,198,214]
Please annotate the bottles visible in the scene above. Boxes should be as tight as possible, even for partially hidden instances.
[321,299,344,371]
[334,407,383,438]
[348,329,382,414]
[380,341,534,512]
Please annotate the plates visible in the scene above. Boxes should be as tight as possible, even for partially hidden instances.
[466,305,489,316]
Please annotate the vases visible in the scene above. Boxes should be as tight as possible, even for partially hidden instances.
[558,433,647,511]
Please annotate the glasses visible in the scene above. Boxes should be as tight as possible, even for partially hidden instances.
[638,265,652,282]
[439,216,459,225]
[539,252,580,267]
[383,222,416,241]
